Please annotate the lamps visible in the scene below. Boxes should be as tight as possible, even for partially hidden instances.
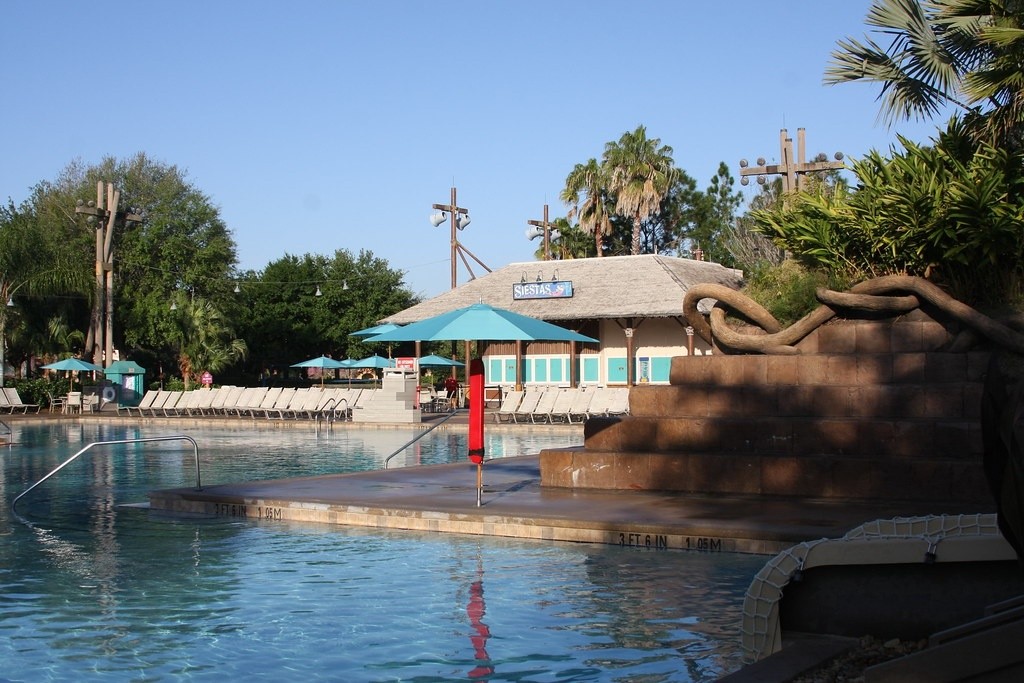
[552,268,559,282]
[536,270,543,283]
[521,270,528,284]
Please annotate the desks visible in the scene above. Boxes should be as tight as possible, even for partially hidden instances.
[59,396,83,413]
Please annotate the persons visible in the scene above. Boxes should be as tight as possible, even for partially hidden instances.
[445,374,461,410]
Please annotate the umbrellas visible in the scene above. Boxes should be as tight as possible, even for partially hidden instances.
[289,353,396,389]
[363,297,600,509]
[350,323,402,337]
[418,354,466,385]
[39,356,104,392]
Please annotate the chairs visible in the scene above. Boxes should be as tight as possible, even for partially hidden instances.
[118,385,457,421]
[0,388,13,413]
[494,385,630,425]
[3,388,40,414]
[66,392,95,414]
[48,392,63,414]
